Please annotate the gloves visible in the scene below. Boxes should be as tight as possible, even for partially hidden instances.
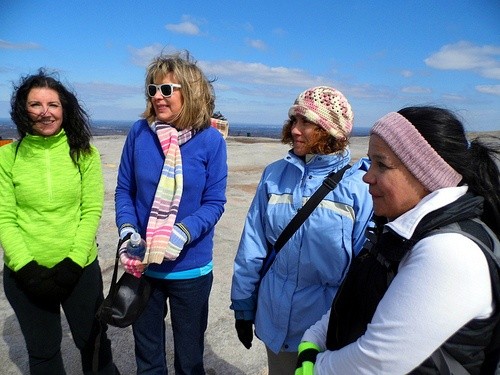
[295,343,321,375]
[164,225,188,260]
[119,227,147,278]
[235,319,254,349]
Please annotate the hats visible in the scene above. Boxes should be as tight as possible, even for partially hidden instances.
[288,86,354,140]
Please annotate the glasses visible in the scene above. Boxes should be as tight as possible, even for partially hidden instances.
[147,83,181,97]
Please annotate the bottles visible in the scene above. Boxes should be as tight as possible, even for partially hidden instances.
[127,233,147,275]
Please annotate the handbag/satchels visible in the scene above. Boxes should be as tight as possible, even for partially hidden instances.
[98,232,150,328]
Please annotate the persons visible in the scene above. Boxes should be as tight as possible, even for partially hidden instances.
[0,66,120,375]
[292,104,500,375]
[114,51,228,375]
[230,85,374,375]
